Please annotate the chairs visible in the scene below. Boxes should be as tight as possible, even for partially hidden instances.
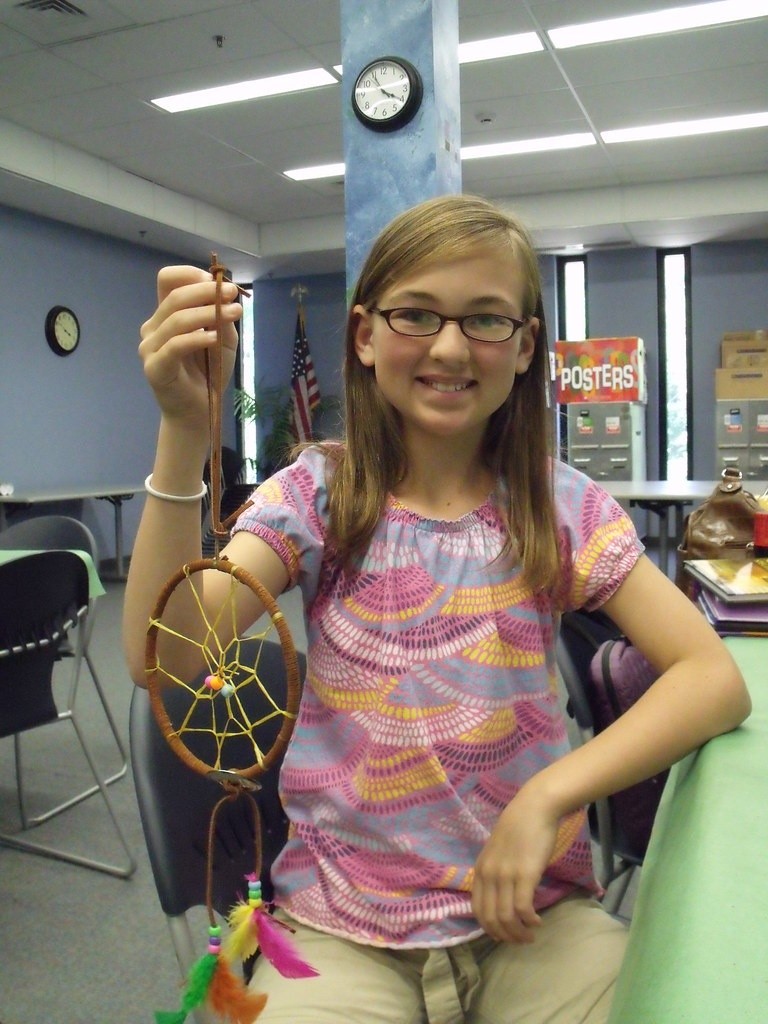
[1,550,138,879]
[201,447,261,558]
[129,639,309,1024]
[557,607,671,924]
[0,516,133,830]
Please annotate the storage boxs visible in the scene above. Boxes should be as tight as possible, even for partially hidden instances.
[721,327,768,368]
[554,337,647,403]
[715,368,768,401]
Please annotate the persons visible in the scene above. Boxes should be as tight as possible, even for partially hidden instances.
[121,195,753,1024]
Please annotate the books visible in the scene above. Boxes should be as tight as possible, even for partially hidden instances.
[683,558,768,637]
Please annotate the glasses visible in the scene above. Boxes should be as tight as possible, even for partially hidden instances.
[369,306,529,342]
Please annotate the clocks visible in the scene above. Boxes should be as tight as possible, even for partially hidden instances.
[353,56,423,130]
[44,305,81,357]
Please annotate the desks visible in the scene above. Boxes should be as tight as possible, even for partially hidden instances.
[592,479,768,577]
[600,625,768,1024]
[0,488,147,581]
[0,549,107,599]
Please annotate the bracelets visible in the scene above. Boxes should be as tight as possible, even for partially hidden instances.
[144,473,209,502]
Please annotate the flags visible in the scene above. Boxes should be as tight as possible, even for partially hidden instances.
[289,312,322,443]
